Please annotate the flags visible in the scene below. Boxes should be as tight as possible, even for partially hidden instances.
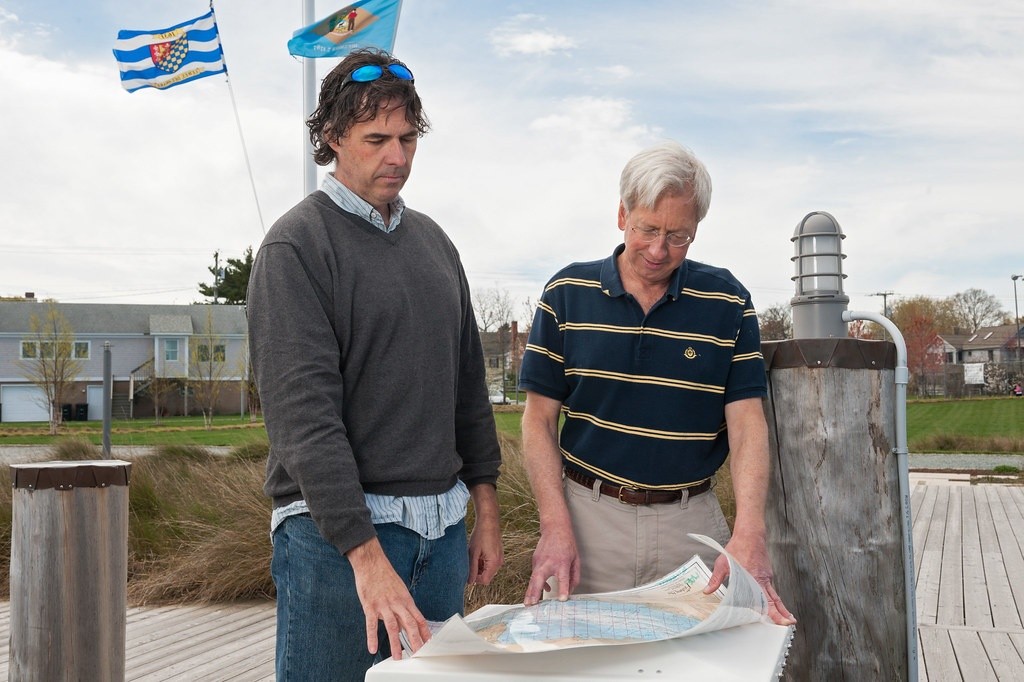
[113,10,226,92]
[287,0,402,58]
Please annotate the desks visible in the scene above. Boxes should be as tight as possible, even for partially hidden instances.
[364,603,796,682]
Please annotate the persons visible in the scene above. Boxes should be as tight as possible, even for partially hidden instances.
[246,49,505,682]
[1014,384,1022,399]
[520,142,798,628]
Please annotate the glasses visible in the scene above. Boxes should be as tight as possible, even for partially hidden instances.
[339,64,414,92]
[628,211,698,248]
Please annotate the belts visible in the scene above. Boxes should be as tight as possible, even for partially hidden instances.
[562,466,711,505]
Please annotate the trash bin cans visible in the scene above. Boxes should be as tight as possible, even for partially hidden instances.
[62,404,71,421]
[75,403,87,421]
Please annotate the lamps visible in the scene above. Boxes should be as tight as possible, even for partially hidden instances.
[790,211,850,338]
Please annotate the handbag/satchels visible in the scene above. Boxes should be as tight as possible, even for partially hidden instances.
[1016,391,1022,394]
